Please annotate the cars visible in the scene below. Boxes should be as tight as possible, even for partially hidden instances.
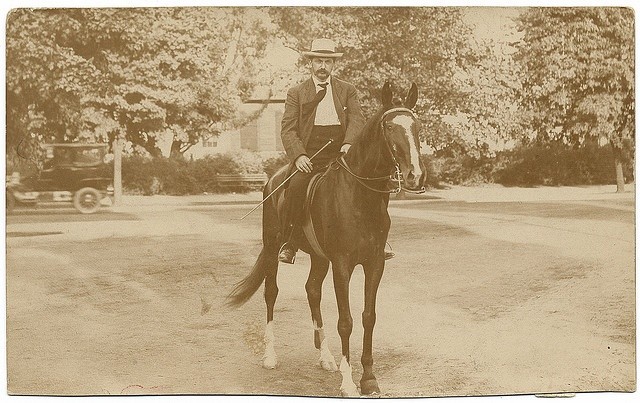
[6,142,112,213]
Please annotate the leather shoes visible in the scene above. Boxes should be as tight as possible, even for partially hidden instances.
[278,248,293,263]
[384,250,395,258]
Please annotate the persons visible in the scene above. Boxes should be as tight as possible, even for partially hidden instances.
[277,37,397,264]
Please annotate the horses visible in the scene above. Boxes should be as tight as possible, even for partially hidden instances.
[223,81,428,398]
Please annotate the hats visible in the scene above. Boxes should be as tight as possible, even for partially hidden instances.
[304,38,344,58]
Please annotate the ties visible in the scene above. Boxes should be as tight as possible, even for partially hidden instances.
[302,83,328,115]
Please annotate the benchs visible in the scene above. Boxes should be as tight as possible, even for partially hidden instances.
[217,171,269,192]
